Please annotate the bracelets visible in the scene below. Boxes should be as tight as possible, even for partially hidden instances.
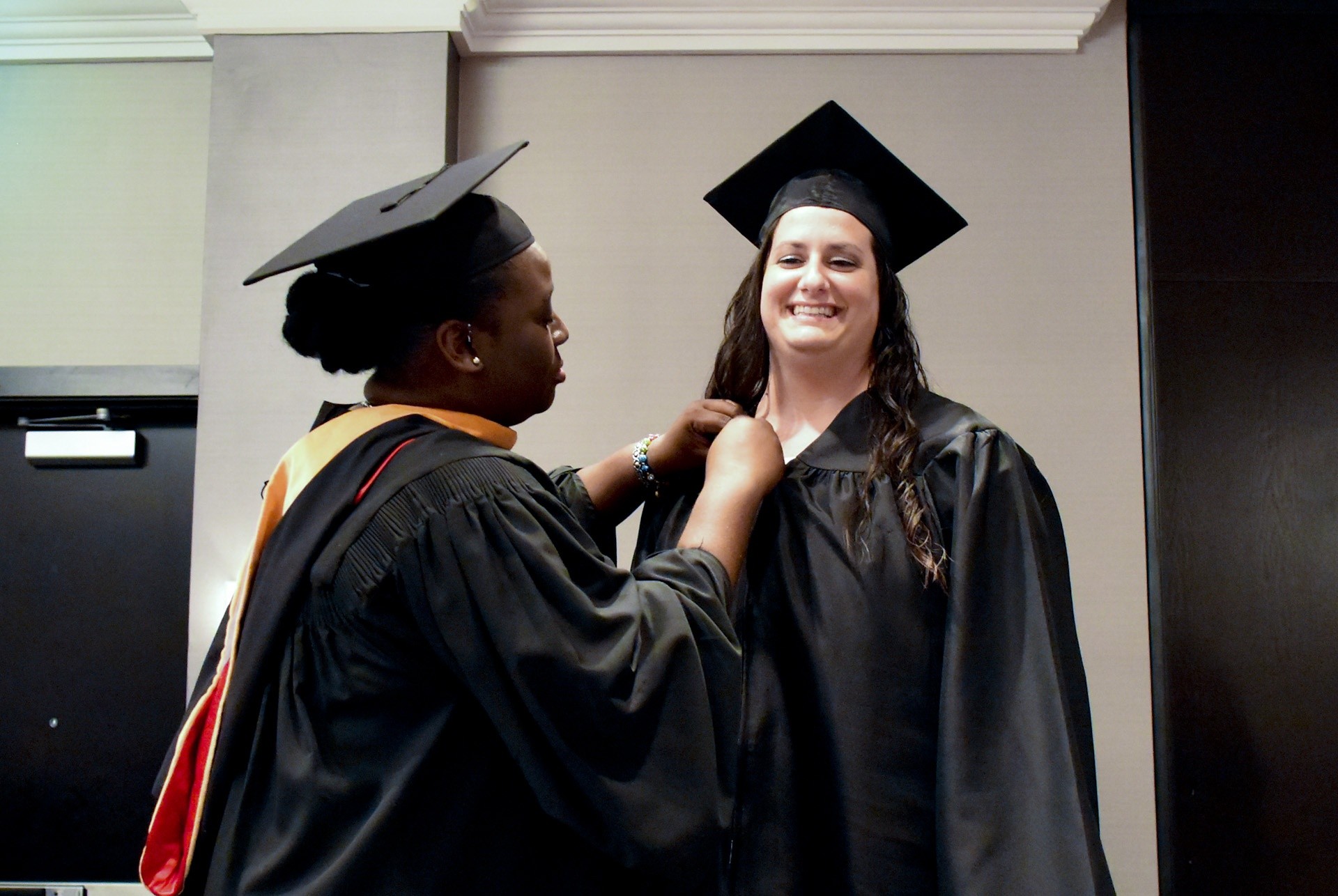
[631,434,666,494]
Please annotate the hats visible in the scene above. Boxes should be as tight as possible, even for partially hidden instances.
[242,141,534,306]
[702,99,970,280]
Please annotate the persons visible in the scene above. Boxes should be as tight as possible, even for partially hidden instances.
[625,166,1117,896]
[135,192,787,895]
